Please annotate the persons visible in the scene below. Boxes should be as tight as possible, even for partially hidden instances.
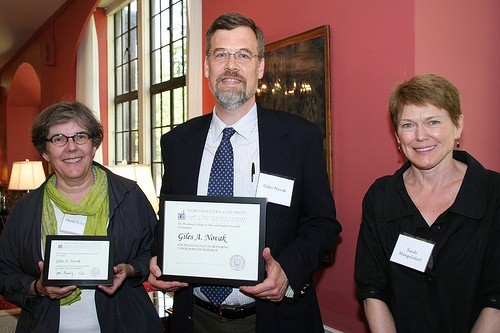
[149,13,343,333]
[0,101,164,333]
[353,75,500,333]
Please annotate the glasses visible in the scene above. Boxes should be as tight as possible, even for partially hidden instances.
[44,132,95,147]
[207,48,260,61]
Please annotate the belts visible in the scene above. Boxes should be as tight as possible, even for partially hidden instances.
[192,294,257,320]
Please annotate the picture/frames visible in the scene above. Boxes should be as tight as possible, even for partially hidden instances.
[255,24,333,192]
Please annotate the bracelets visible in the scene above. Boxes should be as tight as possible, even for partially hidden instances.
[33,280,45,297]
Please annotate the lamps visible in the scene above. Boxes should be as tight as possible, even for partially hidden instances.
[7,158,46,193]
[105,159,158,217]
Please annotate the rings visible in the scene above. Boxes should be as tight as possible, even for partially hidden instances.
[265,296,268,300]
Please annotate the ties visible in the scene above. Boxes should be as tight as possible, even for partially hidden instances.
[200,128,236,309]
